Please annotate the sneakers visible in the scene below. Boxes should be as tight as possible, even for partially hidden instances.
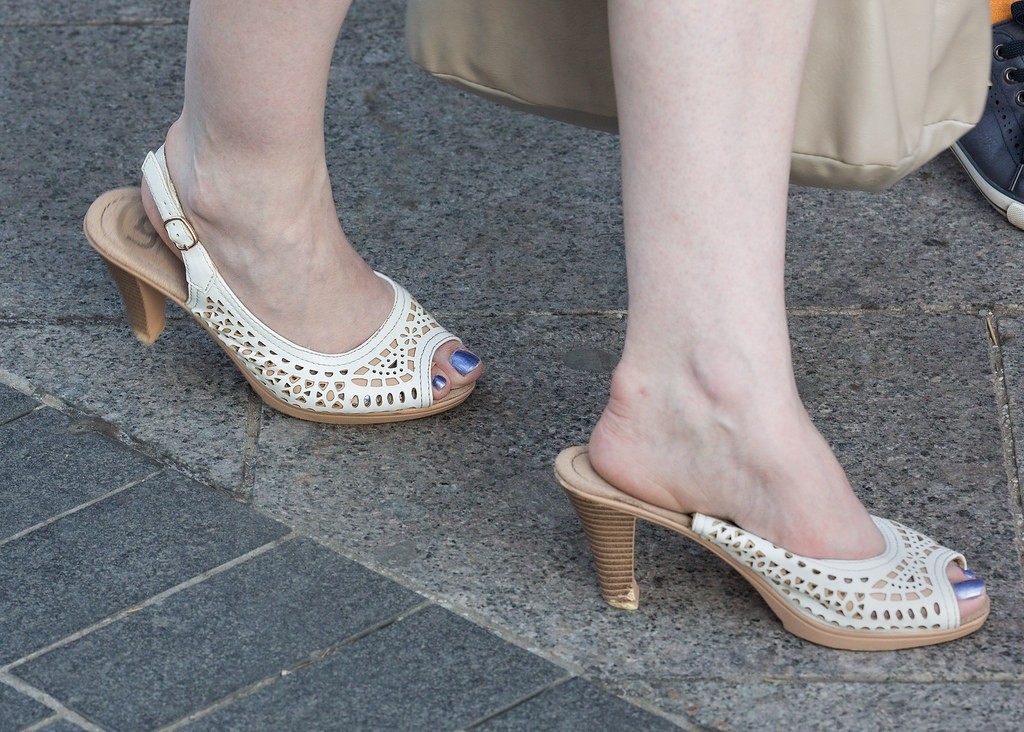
[951,11,1024,231]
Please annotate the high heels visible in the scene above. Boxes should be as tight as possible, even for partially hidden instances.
[83,144,476,423]
[553,440,992,648]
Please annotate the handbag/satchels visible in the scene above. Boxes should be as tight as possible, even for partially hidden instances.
[406,0,996,190]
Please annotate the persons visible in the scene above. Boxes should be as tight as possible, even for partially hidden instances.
[82,0,989,652]
